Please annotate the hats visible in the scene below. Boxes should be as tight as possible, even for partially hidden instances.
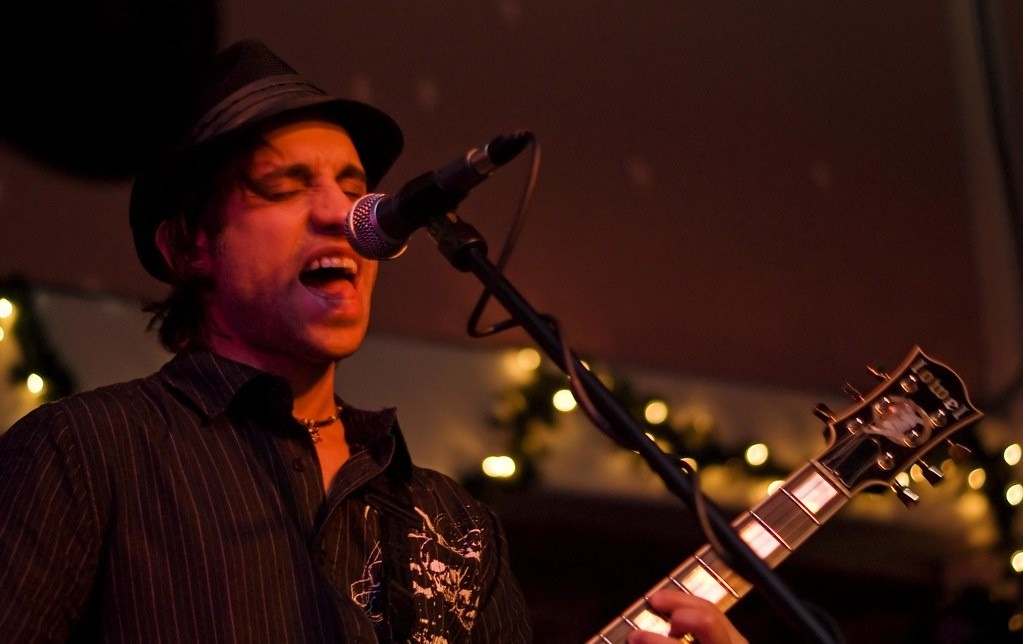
[129,38,405,285]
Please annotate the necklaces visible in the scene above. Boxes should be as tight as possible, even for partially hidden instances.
[292,407,343,441]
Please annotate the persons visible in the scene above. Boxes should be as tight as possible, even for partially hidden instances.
[0,40,749,644]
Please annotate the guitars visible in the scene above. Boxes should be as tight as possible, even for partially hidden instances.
[592,343,983,644]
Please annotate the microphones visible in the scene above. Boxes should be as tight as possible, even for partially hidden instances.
[344,128,530,261]
